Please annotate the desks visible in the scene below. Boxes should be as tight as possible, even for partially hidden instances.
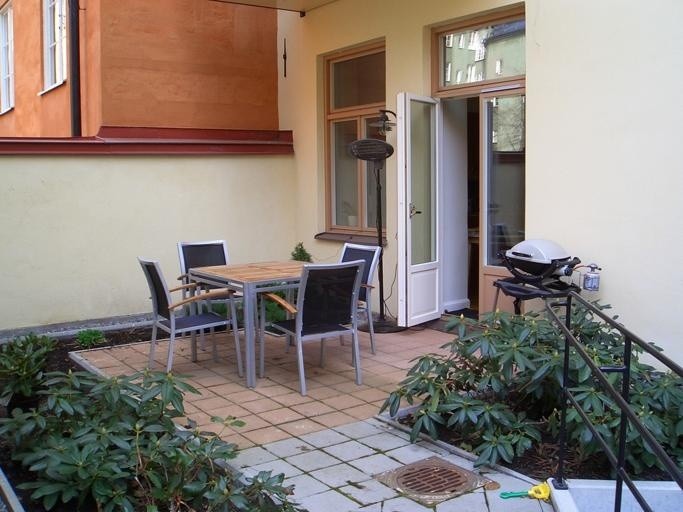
[187,259,318,388]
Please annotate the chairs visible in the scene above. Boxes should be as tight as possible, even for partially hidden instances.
[260,260,366,396]
[319,243,381,367]
[136,256,244,379]
[177,240,243,351]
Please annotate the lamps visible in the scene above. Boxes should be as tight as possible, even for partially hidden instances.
[347,139,408,334]
[368,109,396,137]
